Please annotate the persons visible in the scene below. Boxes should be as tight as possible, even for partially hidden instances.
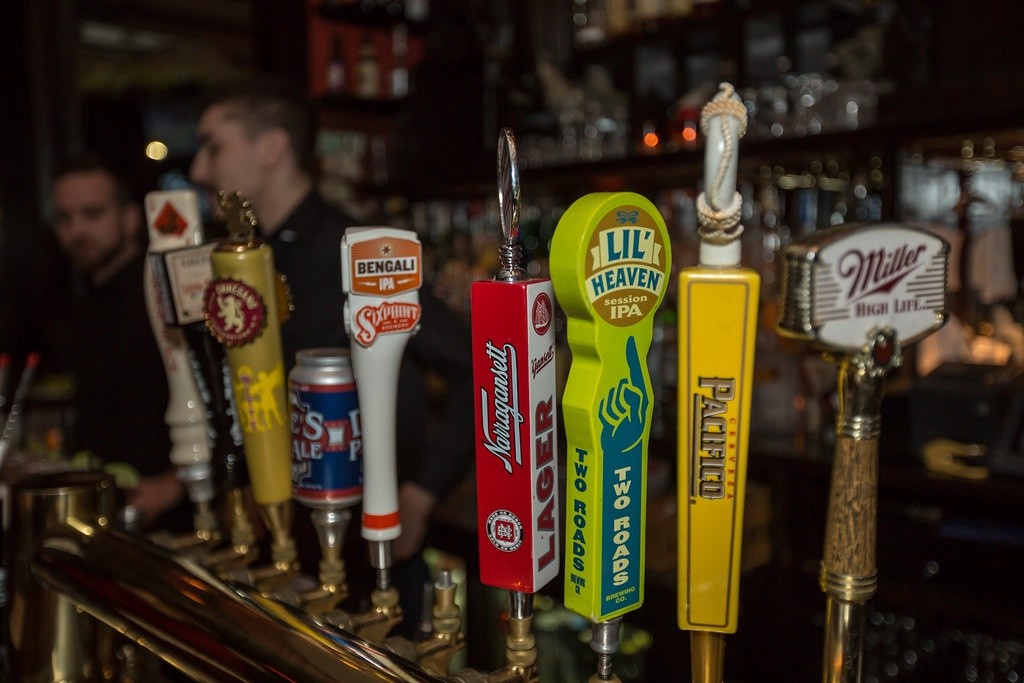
[0,160,197,537]
[124,83,474,642]
